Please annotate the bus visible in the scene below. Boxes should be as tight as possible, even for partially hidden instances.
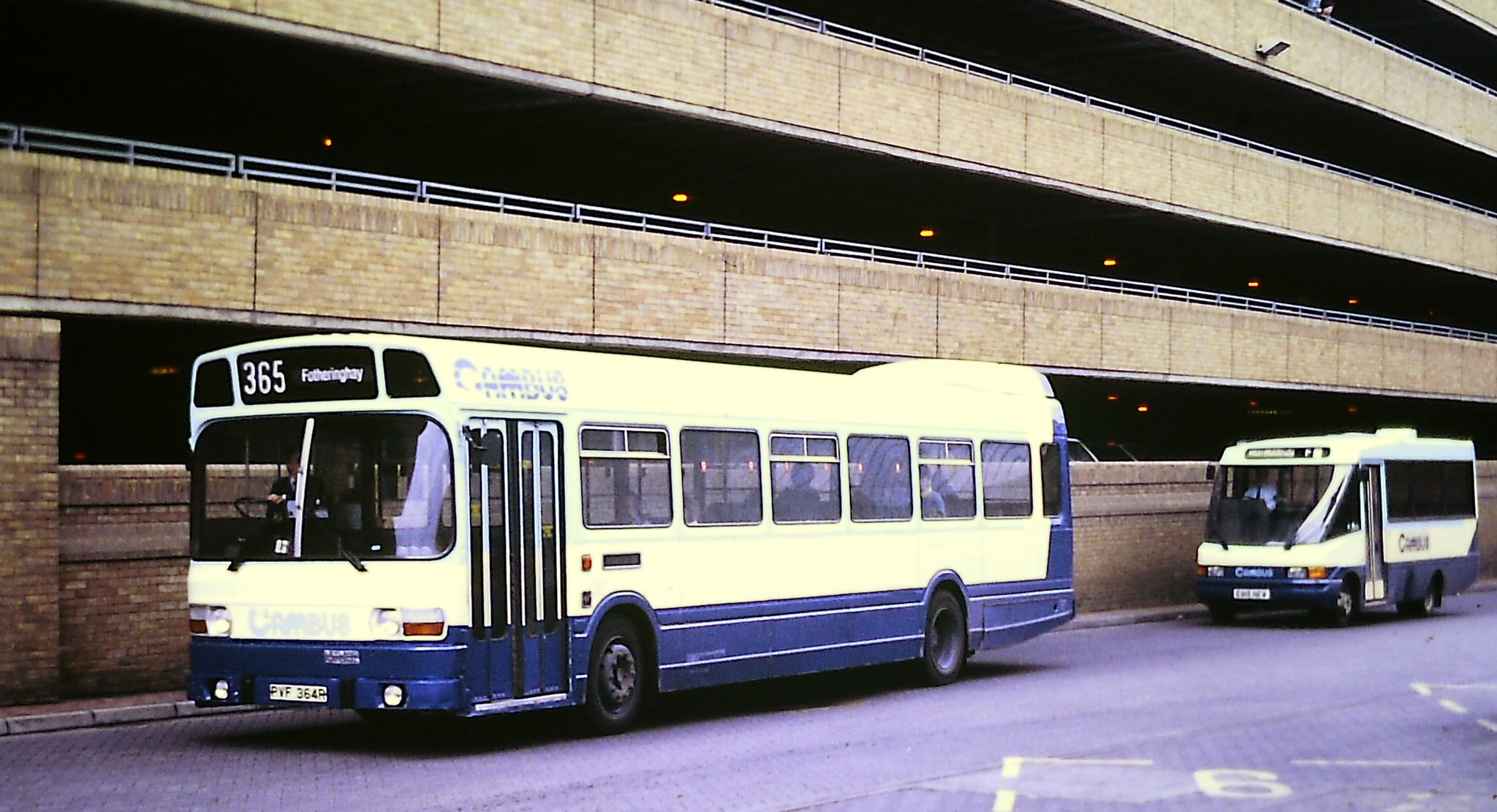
[185,334,1075,739]
[1191,428,1479,627]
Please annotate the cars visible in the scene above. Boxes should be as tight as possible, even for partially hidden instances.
[1067,438,1137,463]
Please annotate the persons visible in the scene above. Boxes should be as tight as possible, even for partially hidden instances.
[1244,469,1278,510]
[617,491,649,525]
[685,497,695,522]
[266,453,325,519]
[920,466,945,518]
